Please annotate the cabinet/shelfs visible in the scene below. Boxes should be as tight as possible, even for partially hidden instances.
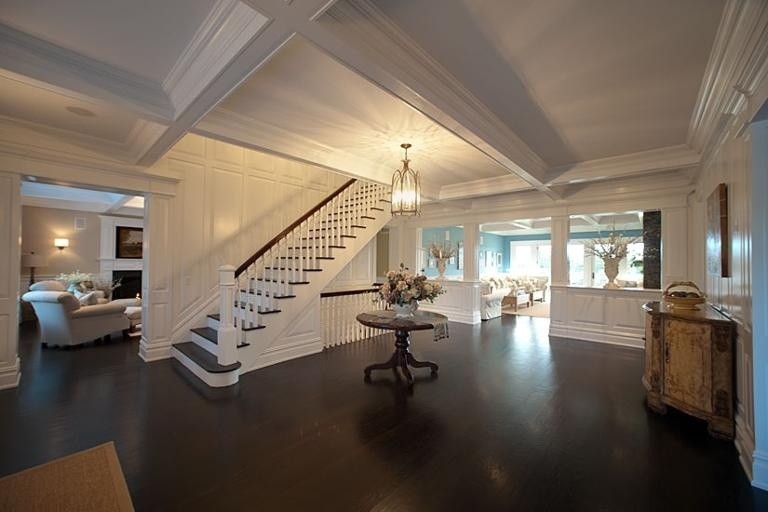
[635,300,740,442]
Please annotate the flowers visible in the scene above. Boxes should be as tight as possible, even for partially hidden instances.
[57,268,88,281]
[422,238,459,259]
[577,222,655,261]
[370,262,442,304]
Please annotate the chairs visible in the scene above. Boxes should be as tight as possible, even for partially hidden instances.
[29,279,108,306]
[21,291,131,352]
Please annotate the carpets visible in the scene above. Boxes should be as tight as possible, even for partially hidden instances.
[0,437,137,511]
[125,323,142,337]
[500,301,550,319]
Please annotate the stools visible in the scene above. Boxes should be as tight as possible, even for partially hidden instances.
[123,307,141,331]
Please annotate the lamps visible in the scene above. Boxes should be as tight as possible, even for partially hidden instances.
[53,236,69,251]
[388,141,421,221]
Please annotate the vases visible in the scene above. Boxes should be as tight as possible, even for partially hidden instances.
[389,301,420,318]
[601,255,622,289]
[66,281,84,292]
[436,258,447,280]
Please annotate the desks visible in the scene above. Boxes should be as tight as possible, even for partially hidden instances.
[356,308,450,396]
[529,289,545,307]
[501,293,531,312]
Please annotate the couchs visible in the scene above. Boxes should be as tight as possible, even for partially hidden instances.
[482,274,550,321]
